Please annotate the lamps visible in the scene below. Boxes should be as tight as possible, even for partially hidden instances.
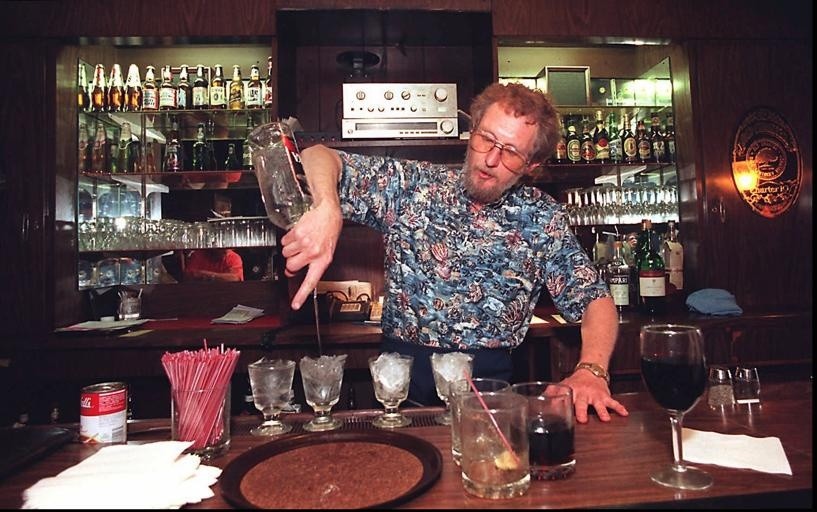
[731,159,759,198]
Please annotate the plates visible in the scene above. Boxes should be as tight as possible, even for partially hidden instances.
[219,432,442,509]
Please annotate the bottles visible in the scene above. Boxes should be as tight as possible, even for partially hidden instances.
[591,219,688,314]
[246,124,309,229]
[76,56,274,112]
[550,109,678,163]
[78,116,258,171]
[706,365,763,407]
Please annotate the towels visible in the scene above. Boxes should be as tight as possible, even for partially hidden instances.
[687,287,741,317]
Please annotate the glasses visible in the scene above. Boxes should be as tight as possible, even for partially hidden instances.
[467,131,529,174]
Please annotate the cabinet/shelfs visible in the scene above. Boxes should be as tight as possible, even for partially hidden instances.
[493,35,706,306]
[45,35,281,319]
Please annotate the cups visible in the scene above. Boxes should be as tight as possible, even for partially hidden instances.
[167,384,232,460]
[119,298,141,322]
[205,217,276,247]
[450,376,578,502]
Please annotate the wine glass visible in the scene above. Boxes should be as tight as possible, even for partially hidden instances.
[429,353,473,426]
[299,356,345,432]
[246,359,295,436]
[637,325,715,491]
[79,215,206,248]
[367,354,414,428]
[560,182,679,225]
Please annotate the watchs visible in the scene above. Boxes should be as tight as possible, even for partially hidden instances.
[571,359,613,387]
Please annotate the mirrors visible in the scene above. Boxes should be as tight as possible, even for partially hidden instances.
[77,46,275,321]
[499,45,682,302]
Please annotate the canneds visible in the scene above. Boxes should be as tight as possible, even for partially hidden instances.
[80,382,128,444]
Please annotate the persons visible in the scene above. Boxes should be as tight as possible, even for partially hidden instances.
[182,248,246,283]
[280,81,630,424]
